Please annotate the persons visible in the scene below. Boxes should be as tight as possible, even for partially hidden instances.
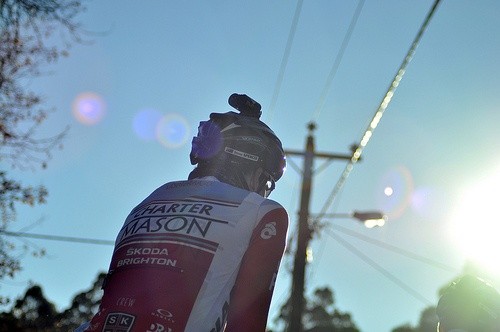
[78,93,290,332]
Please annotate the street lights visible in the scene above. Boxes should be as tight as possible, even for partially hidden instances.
[289,210,384,332]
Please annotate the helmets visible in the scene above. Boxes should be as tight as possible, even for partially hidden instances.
[190,111,286,191]
